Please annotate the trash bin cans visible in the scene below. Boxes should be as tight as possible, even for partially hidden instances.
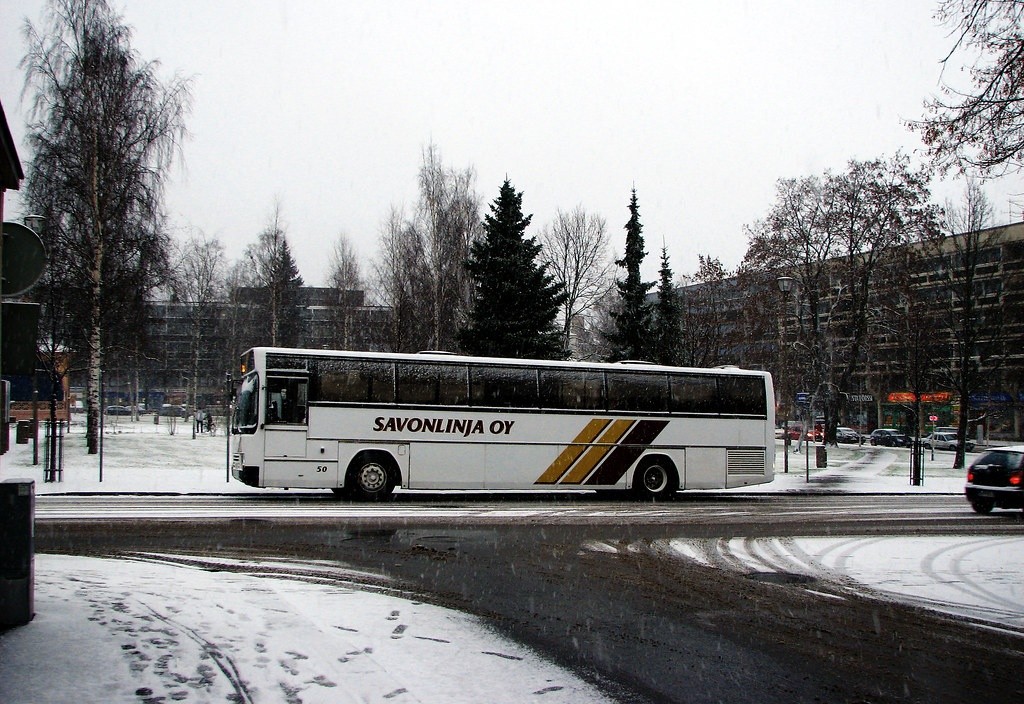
[153,409,159,424]
[16,421,31,444]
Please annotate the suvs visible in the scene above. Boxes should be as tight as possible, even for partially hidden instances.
[965,445,1024,515]
[869,429,913,447]
[782,421,802,431]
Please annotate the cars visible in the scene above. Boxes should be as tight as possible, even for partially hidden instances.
[835,426,859,445]
[789,425,822,441]
[774,424,785,439]
[103,404,188,416]
[921,432,974,452]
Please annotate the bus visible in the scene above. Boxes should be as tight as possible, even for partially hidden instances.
[225,346,776,504]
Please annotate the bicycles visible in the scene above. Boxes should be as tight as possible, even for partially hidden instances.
[201,419,216,434]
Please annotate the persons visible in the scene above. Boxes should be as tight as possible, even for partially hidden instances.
[195,407,215,433]
